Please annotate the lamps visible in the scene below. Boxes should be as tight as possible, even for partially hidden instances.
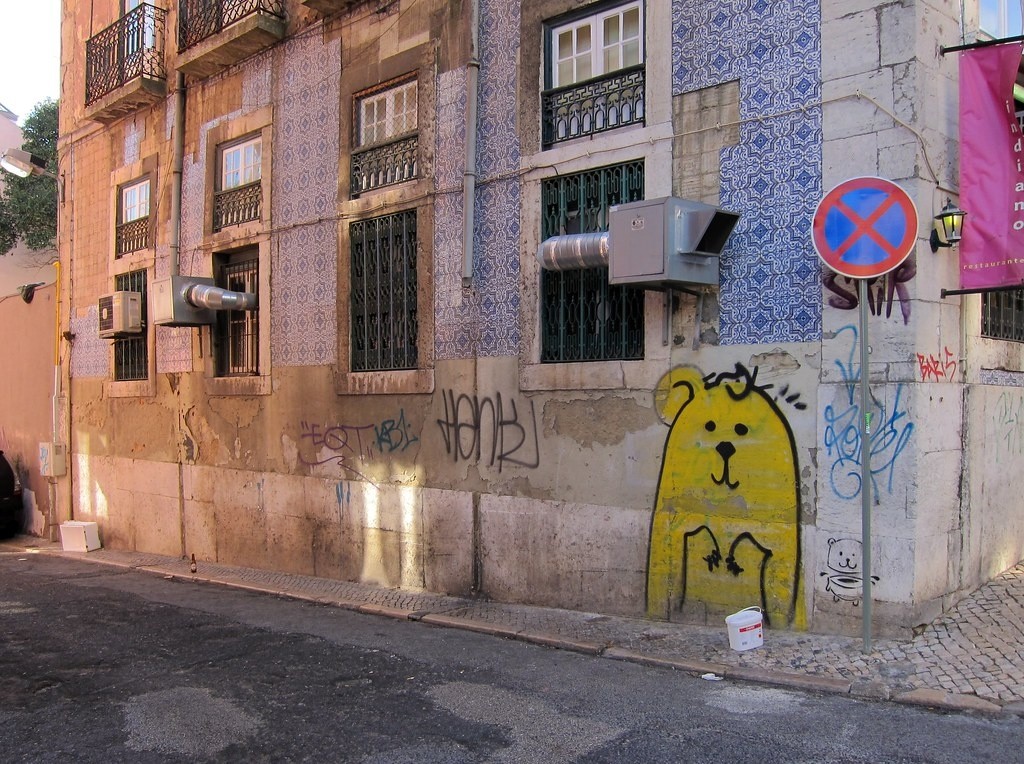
[1,148,66,207]
[929,195,967,252]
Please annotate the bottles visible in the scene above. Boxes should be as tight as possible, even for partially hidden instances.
[190,554,196,572]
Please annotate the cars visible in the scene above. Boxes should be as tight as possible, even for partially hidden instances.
[0,450,23,533]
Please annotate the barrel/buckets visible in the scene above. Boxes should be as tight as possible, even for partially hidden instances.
[725,605,764,651]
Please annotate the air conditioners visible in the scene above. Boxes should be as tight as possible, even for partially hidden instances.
[96,290,142,340]
